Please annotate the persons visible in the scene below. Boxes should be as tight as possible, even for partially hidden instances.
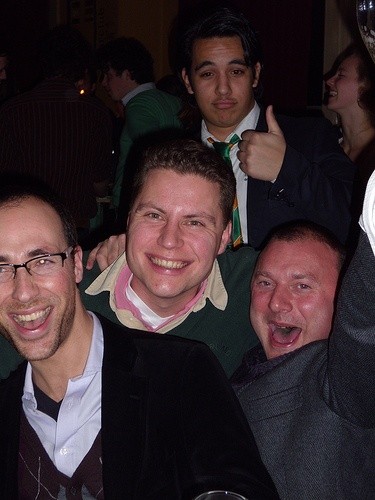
[85,6,356,274]
[0,137,262,378]
[326,48,375,208]
[0,187,280,500]
[0,24,202,249]
[227,169,375,500]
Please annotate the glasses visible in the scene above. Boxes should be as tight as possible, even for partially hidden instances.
[0,246,74,285]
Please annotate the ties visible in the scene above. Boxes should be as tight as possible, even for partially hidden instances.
[206,133,243,251]
